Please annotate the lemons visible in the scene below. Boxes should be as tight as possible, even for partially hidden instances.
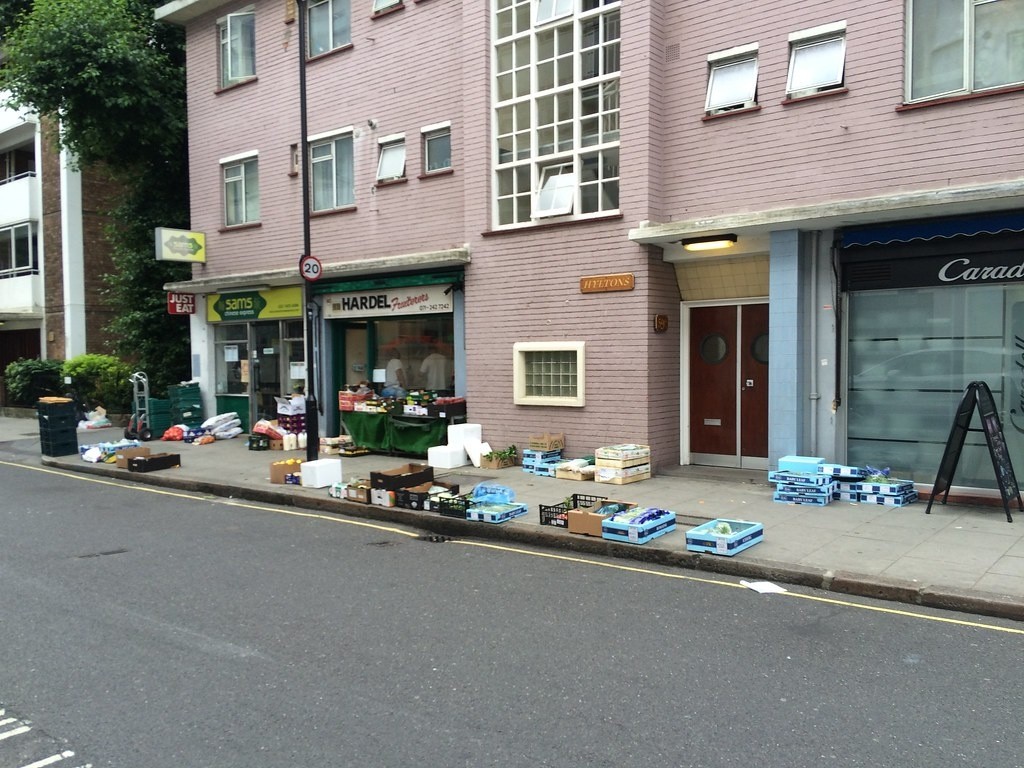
[273,458,301,465]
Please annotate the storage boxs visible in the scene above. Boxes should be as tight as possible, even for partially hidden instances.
[248,389,678,545]
[39,381,213,473]
[768,455,918,506]
[684,518,764,556]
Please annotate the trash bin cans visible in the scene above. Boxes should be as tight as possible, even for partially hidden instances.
[31,369,60,407]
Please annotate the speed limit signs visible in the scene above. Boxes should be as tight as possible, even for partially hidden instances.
[301,256,322,281]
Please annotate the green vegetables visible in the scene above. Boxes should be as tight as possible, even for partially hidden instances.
[483,445,519,461]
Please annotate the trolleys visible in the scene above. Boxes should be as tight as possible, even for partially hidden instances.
[123,371,171,442]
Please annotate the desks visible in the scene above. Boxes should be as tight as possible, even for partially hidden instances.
[340,405,403,458]
[389,415,467,457]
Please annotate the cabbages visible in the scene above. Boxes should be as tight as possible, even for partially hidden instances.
[700,521,732,536]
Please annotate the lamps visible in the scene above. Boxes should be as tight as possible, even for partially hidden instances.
[669,233,738,252]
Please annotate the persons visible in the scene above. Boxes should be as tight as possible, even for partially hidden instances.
[384,347,407,388]
[420,347,451,391]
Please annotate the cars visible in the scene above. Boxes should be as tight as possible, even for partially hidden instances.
[848,347,1022,412]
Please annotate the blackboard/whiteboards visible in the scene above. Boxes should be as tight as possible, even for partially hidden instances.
[931,381,1019,500]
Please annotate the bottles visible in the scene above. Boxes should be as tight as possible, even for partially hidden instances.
[283,429,307,450]
